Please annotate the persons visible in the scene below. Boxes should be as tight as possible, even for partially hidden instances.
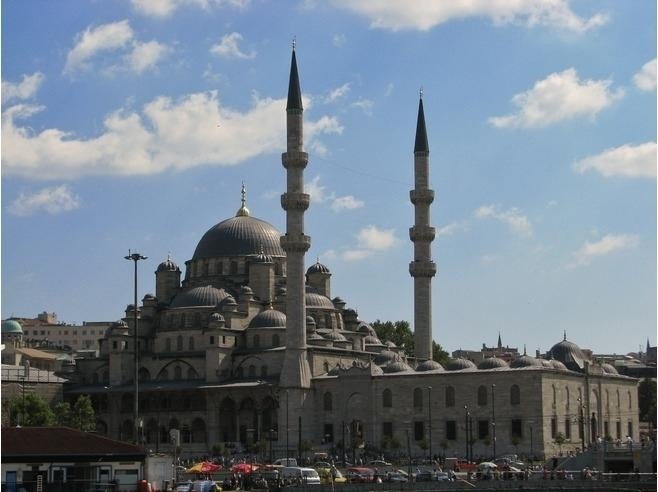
[161,428,656,492]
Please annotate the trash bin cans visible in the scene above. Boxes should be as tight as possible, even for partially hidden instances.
[138,480,147,492]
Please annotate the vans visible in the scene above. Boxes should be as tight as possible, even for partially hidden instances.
[282,466,321,486]
[274,458,297,467]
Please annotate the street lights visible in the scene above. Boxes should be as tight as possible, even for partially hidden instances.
[406,428,413,461]
[18,376,27,427]
[342,421,347,468]
[284,389,291,467]
[529,423,534,454]
[423,384,434,465]
[267,383,276,465]
[577,395,586,452]
[123,252,149,445]
[463,382,497,463]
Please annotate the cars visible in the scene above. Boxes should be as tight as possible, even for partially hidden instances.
[347,460,408,484]
[479,454,525,473]
[312,462,347,484]
